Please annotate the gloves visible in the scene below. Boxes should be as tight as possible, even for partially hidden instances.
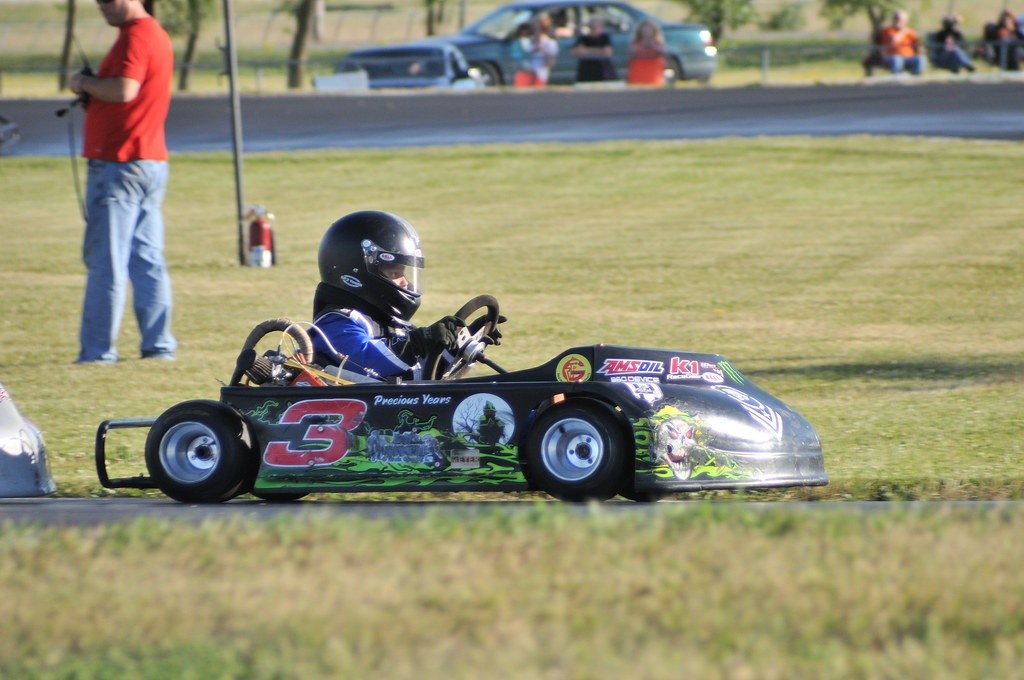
[407,315,467,366]
[467,314,508,346]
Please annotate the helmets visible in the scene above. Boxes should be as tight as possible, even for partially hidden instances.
[318,210,425,322]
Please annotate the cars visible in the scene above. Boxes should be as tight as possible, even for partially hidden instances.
[333,43,485,91]
[434,0,717,89]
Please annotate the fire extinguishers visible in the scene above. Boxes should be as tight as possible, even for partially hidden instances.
[243,204,278,268]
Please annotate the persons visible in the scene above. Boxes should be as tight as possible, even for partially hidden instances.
[930,16,975,73]
[509,15,559,88]
[881,10,926,75]
[984,11,1024,71]
[301,210,507,386]
[67,0,177,363]
[571,19,619,81]
[625,20,670,83]
